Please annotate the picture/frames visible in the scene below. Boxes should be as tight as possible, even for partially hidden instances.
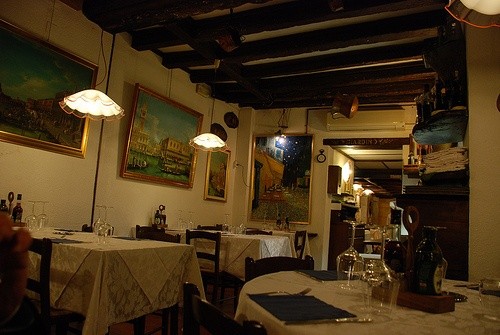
[119,83,204,190]
[0,18,99,159]
[248,134,315,224]
[203,150,232,202]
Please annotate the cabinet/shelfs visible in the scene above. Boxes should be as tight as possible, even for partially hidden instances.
[328,210,365,271]
[396,193,469,281]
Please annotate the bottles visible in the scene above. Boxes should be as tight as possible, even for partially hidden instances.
[285,218,289,226]
[12,193,23,224]
[276,214,281,225]
[0,199,8,213]
[414,226,448,295]
[154,206,166,225]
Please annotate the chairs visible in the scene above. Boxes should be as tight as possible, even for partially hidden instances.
[24,224,314,335]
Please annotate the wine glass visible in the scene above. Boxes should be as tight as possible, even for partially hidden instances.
[223,214,246,235]
[335,220,363,299]
[25,199,49,230]
[95,204,113,246]
[176,210,194,231]
[363,224,405,321]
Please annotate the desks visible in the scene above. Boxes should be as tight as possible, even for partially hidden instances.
[13,227,208,335]
[165,231,293,282]
[234,271,500,335]
[272,231,311,260]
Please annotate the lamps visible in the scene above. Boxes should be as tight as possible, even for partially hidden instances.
[188,95,228,153]
[59,29,125,122]
